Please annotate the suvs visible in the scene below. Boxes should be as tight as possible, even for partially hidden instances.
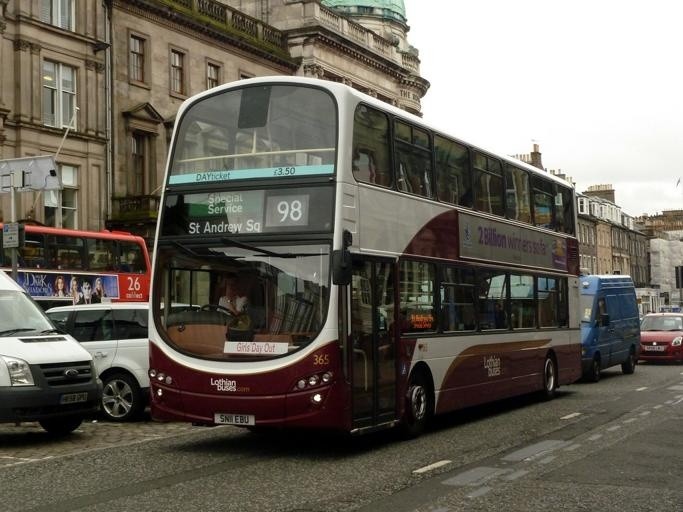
[44,300,200,424]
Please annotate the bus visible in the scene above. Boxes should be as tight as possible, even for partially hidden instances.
[149,74,583,438]
[1,223,152,302]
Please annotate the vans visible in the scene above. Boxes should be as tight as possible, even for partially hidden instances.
[0,269,103,436]
[579,275,641,383]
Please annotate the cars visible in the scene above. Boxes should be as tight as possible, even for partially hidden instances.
[641,313,683,363]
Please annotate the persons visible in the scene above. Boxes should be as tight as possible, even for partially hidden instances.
[74,278,100,304]
[92,278,106,297]
[51,276,67,296]
[67,277,79,297]
[213,277,250,316]
